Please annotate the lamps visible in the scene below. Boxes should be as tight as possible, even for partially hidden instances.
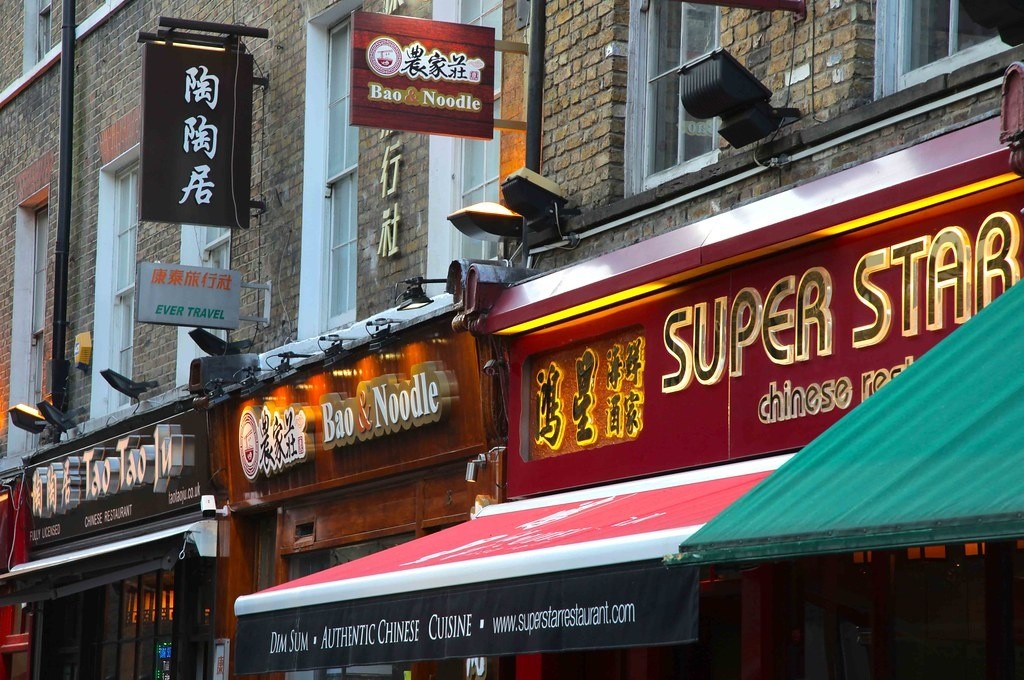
[7,405,51,435]
[445,204,523,243]
[100,369,157,399]
[395,277,448,311]
[232,367,275,399]
[679,49,803,147]
[37,403,84,434]
[366,319,402,352]
[317,334,357,370]
[501,169,569,231]
[265,352,312,385]
[465,451,486,484]
[188,328,249,353]
[202,379,237,408]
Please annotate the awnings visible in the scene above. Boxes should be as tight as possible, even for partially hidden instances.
[662,276,1024,568]
[234,453,797,677]
